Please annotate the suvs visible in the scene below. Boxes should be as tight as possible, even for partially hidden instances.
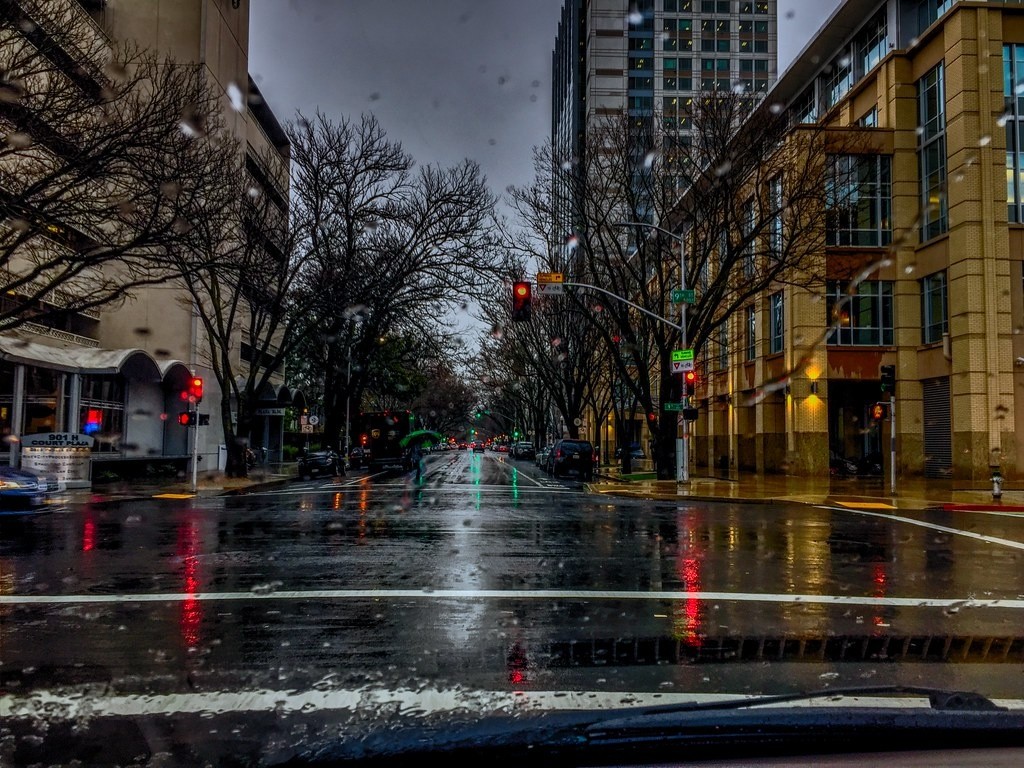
[514,441,535,462]
[546,438,595,482]
[472,440,485,453]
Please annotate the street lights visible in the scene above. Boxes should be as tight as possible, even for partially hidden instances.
[611,221,693,487]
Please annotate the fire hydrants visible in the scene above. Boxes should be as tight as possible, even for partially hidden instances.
[989,471,1006,499]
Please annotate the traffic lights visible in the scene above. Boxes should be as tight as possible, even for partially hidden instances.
[179,411,198,426]
[881,365,894,394]
[686,372,695,396]
[511,281,532,321]
[190,377,202,405]
[872,403,884,423]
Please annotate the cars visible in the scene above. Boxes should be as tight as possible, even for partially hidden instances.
[535,447,552,470]
[488,443,516,458]
[423,442,467,456]
[350,447,371,471]
[298,447,347,482]
[0,464,59,525]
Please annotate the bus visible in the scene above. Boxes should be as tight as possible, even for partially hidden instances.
[359,409,422,475]
